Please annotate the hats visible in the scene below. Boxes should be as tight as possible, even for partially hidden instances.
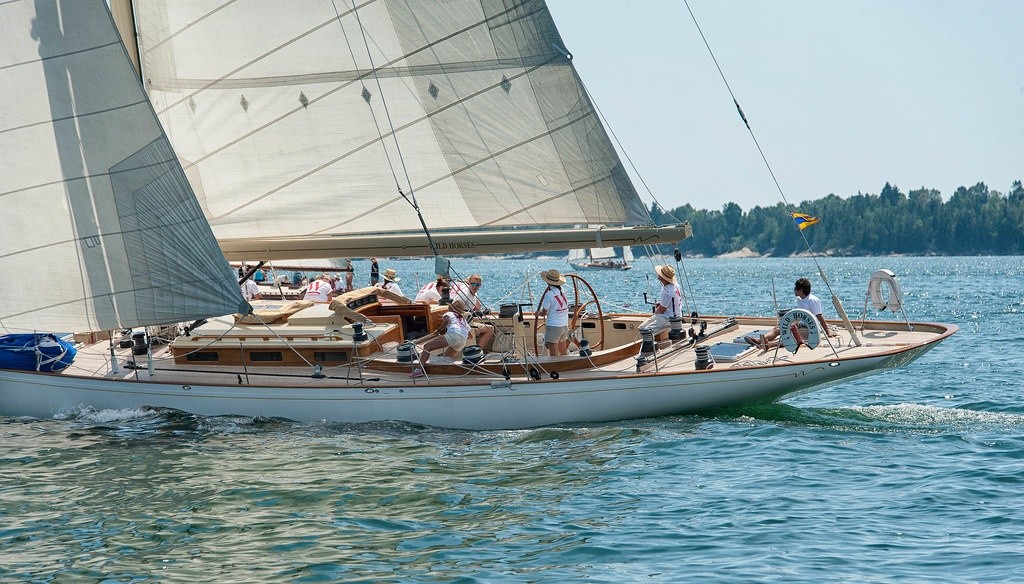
[334,272,342,280]
[445,300,465,315]
[541,269,566,285]
[378,268,400,282]
[655,264,676,284]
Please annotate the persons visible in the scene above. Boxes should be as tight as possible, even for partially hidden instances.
[413,273,494,351]
[637,264,682,335]
[406,300,472,379]
[744,277,838,351]
[371,257,379,287]
[236,258,354,302]
[535,269,569,356]
[374,269,403,302]
[303,274,333,301]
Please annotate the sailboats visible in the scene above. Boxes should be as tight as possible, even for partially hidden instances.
[565,223,635,271]
[230,260,357,299]
[0,0,958,429]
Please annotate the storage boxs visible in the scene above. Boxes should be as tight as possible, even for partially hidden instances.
[74,330,112,343]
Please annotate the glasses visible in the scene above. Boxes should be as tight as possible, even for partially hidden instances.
[470,282,481,287]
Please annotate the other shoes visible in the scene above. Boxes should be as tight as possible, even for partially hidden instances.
[759,334,769,352]
[744,336,761,349]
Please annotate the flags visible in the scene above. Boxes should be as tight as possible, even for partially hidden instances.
[793,212,820,231]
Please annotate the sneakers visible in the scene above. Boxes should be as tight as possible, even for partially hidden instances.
[408,369,423,378]
